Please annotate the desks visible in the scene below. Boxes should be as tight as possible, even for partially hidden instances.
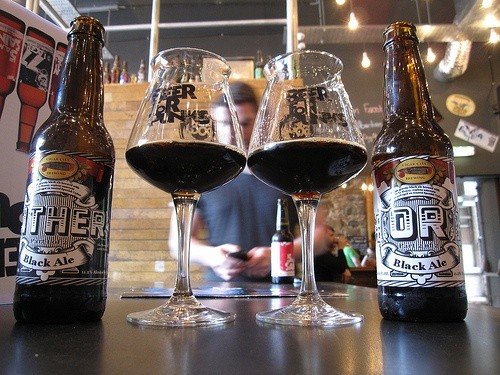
[0,282,500,375]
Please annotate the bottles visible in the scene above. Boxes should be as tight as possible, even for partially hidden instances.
[271,198,296,284]
[102,54,145,84]
[13,16,115,325]
[254,49,265,79]
[371,21,468,323]
[49,42,69,112]
[0,8,26,119]
[16,27,56,154]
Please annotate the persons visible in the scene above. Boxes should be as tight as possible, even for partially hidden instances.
[313,226,351,284]
[362,232,376,267]
[168,82,326,282]
[342,243,360,267]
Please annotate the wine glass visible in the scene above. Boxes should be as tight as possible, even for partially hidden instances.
[247,51,368,327]
[125,47,246,327]
